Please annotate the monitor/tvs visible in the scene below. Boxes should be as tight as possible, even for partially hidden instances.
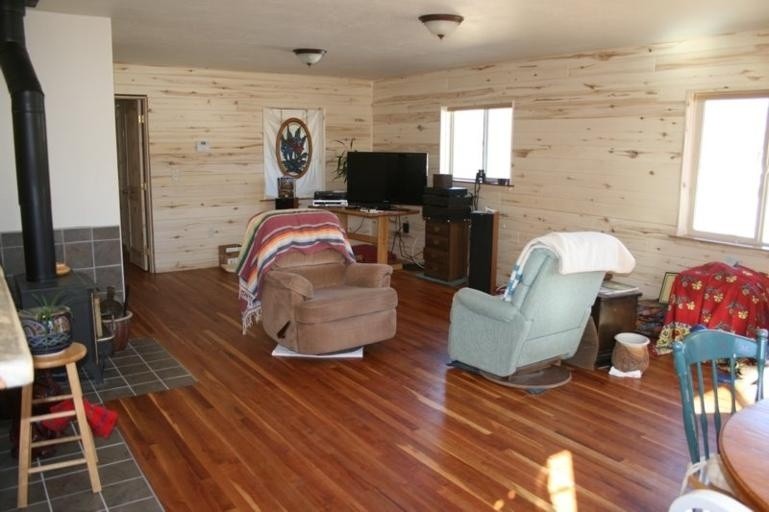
[347,151,427,211]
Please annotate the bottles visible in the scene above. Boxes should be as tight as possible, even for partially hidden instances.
[475,169,486,184]
[614,330,652,373]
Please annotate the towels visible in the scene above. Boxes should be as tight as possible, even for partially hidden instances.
[43,397,119,441]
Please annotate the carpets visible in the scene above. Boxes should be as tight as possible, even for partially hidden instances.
[0,336,199,512]
[416,269,469,287]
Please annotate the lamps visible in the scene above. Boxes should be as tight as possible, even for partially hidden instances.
[293,48,327,66]
[418,14,464,40]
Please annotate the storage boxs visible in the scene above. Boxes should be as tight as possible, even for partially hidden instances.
[218,243,242,270]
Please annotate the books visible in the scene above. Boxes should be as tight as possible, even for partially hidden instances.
[599,279,639,294]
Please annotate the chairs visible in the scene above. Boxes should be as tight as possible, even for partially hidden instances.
[236,209,398,358]
[673,329,769,511]
[446,231,636,394]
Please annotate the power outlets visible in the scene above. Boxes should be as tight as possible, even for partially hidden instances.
[403,223,409,234]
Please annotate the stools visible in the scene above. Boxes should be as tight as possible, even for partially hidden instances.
[6,342,103,509]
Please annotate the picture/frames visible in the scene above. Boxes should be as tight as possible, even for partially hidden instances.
[658,272,680,304]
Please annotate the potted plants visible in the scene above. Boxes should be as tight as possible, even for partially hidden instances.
[17,292,79,356]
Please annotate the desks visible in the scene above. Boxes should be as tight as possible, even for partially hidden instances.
[718,396,768,512]
[308,205,420,271]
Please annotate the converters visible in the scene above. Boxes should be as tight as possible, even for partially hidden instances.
[403,223,409,233]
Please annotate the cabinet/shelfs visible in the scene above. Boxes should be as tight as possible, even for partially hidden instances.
[423,219,465,282]
[563,278,643,371]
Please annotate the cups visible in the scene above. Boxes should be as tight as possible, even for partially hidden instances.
[277,176,296,198]
